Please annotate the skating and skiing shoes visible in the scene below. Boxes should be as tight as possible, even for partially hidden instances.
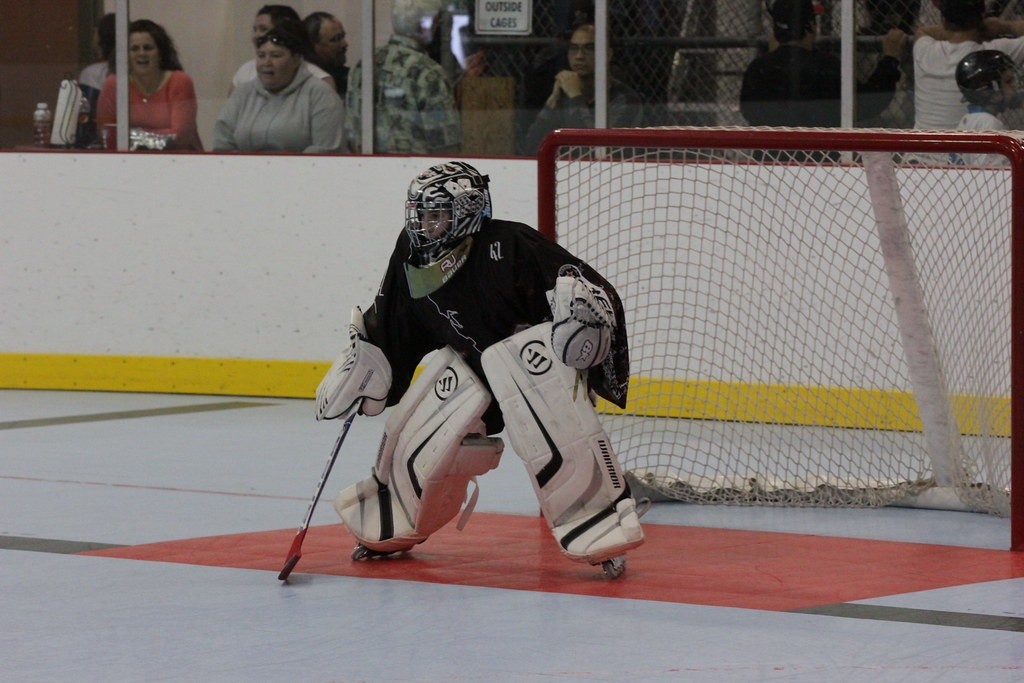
[350,541,417,560]
[591,552,628,579]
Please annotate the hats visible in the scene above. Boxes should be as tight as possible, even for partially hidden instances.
[931,0,985,22]
[773,0,824,29]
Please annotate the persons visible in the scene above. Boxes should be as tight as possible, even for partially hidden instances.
[78,13,116,148]
[215,18,350,154]
[948,49,1024,167]
[740,0,907,161]
[903,0,1024,164]
[316,162,645,578]
[96,19,206,150]
[303,12,350,103]
[228,5,337,93]
[345,0,464,154]
[521,23,644,161]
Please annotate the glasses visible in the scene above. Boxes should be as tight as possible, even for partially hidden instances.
[567,44,594,56]
[256,35,289,47]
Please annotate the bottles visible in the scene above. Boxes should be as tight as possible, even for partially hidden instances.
[33,103,51,147]
[75,87,91,150]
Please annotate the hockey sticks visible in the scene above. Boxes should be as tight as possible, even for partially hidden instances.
[280,397,362,579]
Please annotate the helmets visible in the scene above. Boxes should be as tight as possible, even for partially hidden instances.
[404,161,492,270]
[393,0,441,43]
[955,49,1024,107]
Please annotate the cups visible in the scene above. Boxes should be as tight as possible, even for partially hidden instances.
[102,124,116,150]
